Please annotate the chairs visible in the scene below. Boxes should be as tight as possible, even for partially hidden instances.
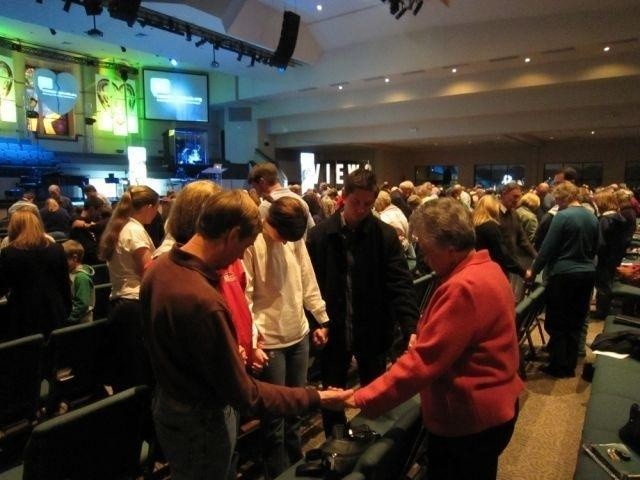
[1,214,640,480]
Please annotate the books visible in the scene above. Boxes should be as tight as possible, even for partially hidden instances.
[590,444,639,479]
[583,444,616,478]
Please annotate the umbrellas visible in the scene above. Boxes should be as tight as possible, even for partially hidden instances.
[538,359,564,379]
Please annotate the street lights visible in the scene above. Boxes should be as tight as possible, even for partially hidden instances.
[616,264,640,284]
[295,423,382,479]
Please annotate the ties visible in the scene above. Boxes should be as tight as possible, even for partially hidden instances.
[143,68,209,124]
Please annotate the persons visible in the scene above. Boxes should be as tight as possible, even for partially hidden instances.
[0,161,640,479]
[331,199,526,480]
[138,195,360,476]
